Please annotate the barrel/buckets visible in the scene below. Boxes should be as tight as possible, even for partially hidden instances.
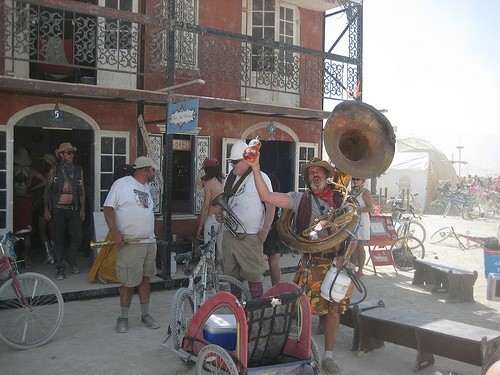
[484,247,500,278]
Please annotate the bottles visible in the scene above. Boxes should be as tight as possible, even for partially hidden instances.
[242,135,262,162]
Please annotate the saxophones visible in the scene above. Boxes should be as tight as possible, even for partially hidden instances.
[89,236,158,250]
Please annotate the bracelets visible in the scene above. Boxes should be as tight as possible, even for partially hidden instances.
[343,254,350,258]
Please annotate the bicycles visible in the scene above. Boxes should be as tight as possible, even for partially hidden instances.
[170,225,252,367]
[390,174,500,272]
[0,229,65,350]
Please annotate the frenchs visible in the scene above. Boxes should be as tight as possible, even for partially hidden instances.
[276,100,396,254]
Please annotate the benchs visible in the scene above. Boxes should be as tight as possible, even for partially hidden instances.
[314,300,500,375]
[411,256,478,304]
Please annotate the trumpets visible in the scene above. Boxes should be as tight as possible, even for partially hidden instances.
[211,191,247,240]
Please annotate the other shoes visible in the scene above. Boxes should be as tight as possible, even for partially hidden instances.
[117,315,128,332]
[322,357,341,375]
[353,272,364,278]
[57,270,64,280]
[71,264,79,274]
[141,314,160,329]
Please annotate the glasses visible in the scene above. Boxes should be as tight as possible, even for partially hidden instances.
[352,178,360,180]
[231,160,242,164]
[63,151,74,154]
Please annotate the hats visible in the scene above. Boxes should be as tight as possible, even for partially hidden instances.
[132,156,160,170]
[303,157,335,183]
[229,141,249,164]
[56,143,78,157]
[201,156,219,167]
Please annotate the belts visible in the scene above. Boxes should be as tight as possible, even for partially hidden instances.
[54,205,80,211]
[304,252,340,258]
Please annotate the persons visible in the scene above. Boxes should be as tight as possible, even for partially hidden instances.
[214,141,284,301]
[455,174,500,202]
[102,156,161,333]
[341,176,373,279]
[193,157,223,269]
[42,142,87,281]
[12,144,54,265]
[242,151,359,375]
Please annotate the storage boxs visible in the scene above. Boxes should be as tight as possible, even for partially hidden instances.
[203,314,237,351]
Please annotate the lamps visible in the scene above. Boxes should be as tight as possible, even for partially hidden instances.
[267,120,278,139]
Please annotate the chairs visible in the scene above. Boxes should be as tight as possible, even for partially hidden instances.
[39,39,80,83]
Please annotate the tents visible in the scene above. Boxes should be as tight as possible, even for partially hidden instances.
[345,135,462,216]
